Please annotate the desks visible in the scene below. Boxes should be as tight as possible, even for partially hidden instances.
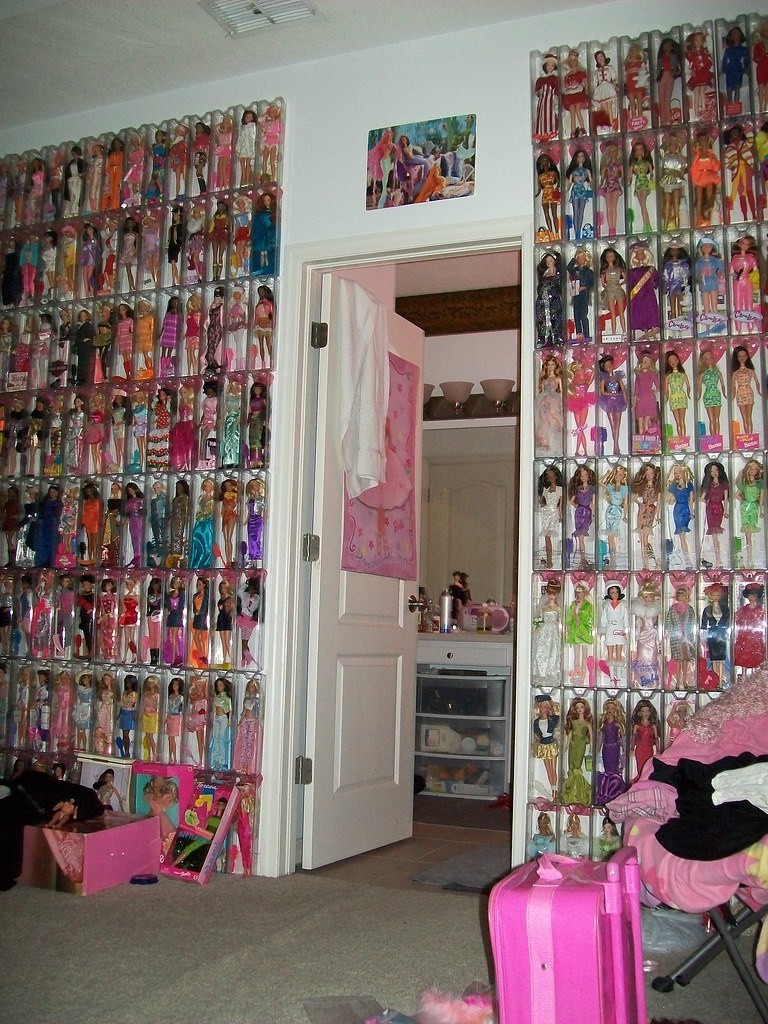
[416,633,515,784]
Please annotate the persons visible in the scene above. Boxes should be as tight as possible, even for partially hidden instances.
[2,100,285,880]
[530,16,766,860]
[449,571,464,629]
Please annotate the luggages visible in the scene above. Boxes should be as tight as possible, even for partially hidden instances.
[486,844,647,1024]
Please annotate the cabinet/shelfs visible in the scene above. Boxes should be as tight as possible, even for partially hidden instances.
[415,675,509,801]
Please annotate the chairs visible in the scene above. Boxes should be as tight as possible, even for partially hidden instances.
[613,665,768,1024]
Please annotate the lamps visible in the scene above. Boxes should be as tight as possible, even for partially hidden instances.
[480,379,514,413]
[438,381,474,415]
[423,383,435,406]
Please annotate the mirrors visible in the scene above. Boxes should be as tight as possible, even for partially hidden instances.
[420,424,517,613]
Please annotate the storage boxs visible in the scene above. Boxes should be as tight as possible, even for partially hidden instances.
[22,812,161,894]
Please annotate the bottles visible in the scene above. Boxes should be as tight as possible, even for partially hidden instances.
[477,603,493,635]
[439,588,453,633]
